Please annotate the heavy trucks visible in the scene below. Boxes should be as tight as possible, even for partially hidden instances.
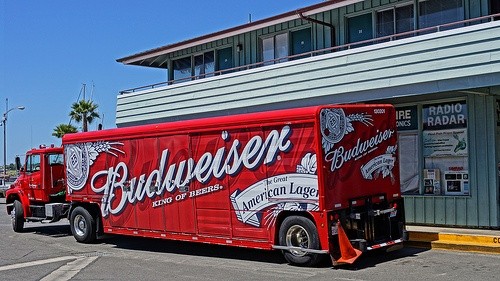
[4,104,409,268]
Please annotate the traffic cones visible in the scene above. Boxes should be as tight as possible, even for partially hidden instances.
[336,218,362,265]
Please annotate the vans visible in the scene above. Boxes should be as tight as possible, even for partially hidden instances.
[0,176,17,197]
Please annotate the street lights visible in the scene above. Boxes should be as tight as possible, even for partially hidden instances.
[0,106,24,178]
